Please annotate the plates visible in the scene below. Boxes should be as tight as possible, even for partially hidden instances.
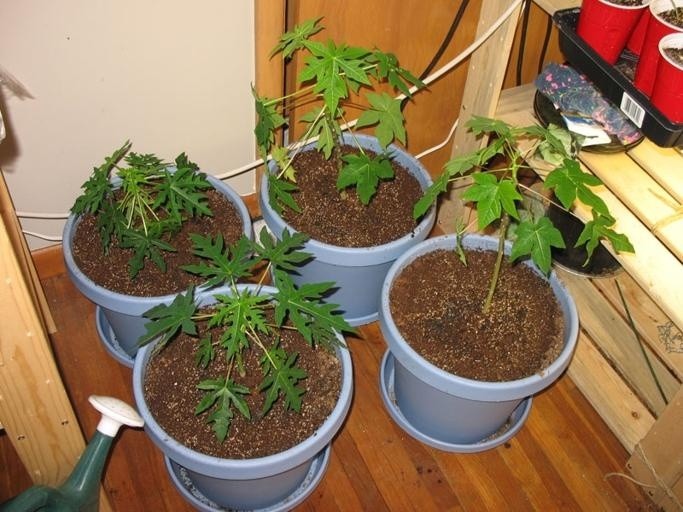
[533,61,645,153]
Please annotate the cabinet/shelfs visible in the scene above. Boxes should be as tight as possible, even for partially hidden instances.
[434,0,683,456]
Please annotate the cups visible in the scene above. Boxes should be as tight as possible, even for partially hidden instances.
[576,0,683,125]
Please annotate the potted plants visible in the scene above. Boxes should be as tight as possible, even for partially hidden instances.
[130,226,365,512]
[376,112,634,445]
[578,0,683,128]
[249,13,437,326]
[61,137,254,359]
